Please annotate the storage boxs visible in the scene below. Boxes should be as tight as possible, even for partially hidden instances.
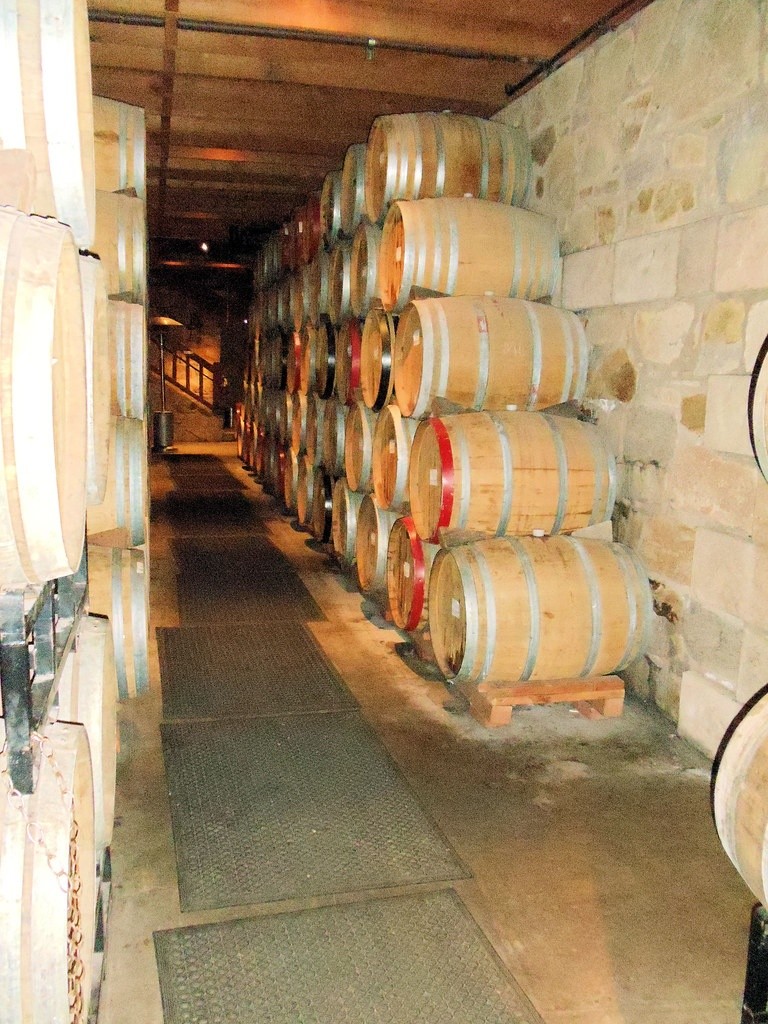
[232,114,649,694]
[712,688,764,906]
[1,0,155,1024]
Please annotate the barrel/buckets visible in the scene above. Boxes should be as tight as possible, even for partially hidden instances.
[0,1,149,1024]
[235,114,655,689]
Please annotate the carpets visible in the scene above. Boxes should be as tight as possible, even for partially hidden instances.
[156,454,541,1024]
